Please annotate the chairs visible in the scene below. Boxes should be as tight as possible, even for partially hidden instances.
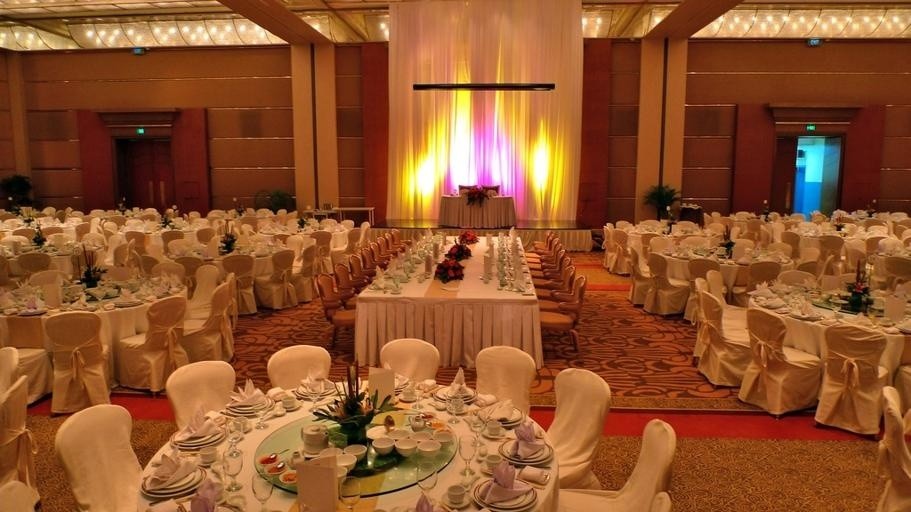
[183,283,235,367]
[342,219,354,229]
[878,386,911,512]
[317,273,355,347]
[644,252,689,316]
[102,221,117,234]
[641,220,660,233]
[18,254,50,283]
[677,221,698,233]
[152,261,184,283]
[712,212,721,217]
[113,244,130,267]
[334,264,368,309]
[535,231,552,243]
[525,244,561,276]
[349,255,376,292]
[41,227,63,241]
[536,236,560,265]
[370,243,390,266]
[44,311,111,413]
[43,207,55,216]
[197,228,215,245]
[607,223,614,231]
[706,223,725,253]
[831,209,911,259]
[535,266,576,310]
[703,213,713,228]
[777,271,816,285]
[104,229,115,249]
[331,230,362,267]
[731,239,754,262]
[12,229,36,243]
[814,326,889,436]
[376,237,398,260]
[13,348,56,405]
[91,217,100,233]
[278,209,287,226]
[697,291,750,388]
[602,226,610,269]
[842,242,866,274]
[120,296,189,399]
[817,255,835,285]
[684,258,727,326]
[391,229,412,249]
[384,233,405,254]
[706,270,746,311]
[240,224,252,236]
[344,222,370,248]
[649,237,674,276]
[536,245,570,296]
[0,255,21,292]
[878,236,903,257]
[768,242,791,258]
[48,233,73,248]
[256,250,297,310]
[103,235,127,265]
[74,223,90,240]
[291,246,318,302]
[885,257,911,288]
[189,211,201,225]
[680,236,710,251]
[72,211,84,222]
[286,211,297,219]
[187,265,219,308]
[693,277,747,362]
[2,236,30,254]
[475,346,536,421]
[539,275,586,355]
[0,375,41,512]
[818,236,846,275]
[378,337,441,382]
[608,230,633,277]
[55,404,145,512]
[236,235,249,247]
[208,235,231,259]
[174,257,204,292]
[528,236,553,256]
[54,210,65,223]
[125,230,148,255]
[482,185,500,195]
[362,248,387,273]
[168,240,191,255]
[559,419,677,512]
[69,250,97,280]
[738,308,822,418]
[834,239,867,274]
[83,233,107,271]
[161,230,184,255]
[286,235,303,262]
[781,232,799,258]
[287,218,300,226]
[190,272,237,328]
[798,261,818,274]
[166,361,236,431]
[766,222,786,242]
[737,219,765,244]
[128,256,160,276]
[627,247,655,305]
[90,210,105,220]
[864,236,887,274]
[459,185,477,195]
[221,254,257,316]
[266,345,332,391]
[112,216,126,230]
[616,221,631,228]
[545,367,612,491]
[30,270,69,285]
[310,231,334,275]
[732,261,781,305]
[0,346,18,392]
[528,251,566,287]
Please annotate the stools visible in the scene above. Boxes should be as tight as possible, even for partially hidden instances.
[303,210,314,218]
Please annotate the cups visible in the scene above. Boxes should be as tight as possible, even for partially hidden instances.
[233,417,248,429]
[199,447,217,462]
[403,388,414,398]
[282,396,296,408]
[486,454,502,467]
[488,420,501,435]
[211,482,224,501]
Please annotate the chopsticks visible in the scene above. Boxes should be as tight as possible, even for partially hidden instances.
[481,468,545,490]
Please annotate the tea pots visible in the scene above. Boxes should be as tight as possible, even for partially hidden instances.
[286,452,304,469]
[300,424,329,454]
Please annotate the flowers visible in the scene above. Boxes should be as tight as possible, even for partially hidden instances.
[312,354,404,435]
[78,243,108,281]
[846,259,871,299]
[33,226,46,243]
[220,219,237,244]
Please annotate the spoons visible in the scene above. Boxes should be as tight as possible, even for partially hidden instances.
[276,461,285,469]
[269,449,290,459]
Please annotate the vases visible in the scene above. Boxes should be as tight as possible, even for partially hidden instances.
[834,224,843,231]
[81,279,99,288]
[849,298,862,310]
[225,244,234,251]
[347,430,365,449]
[718,242,735,259]
[37,242,44,246]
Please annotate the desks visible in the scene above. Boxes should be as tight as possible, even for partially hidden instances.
[440,196,517,228]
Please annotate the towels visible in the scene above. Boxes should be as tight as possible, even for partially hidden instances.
[824,310,872,330]
[757,295,786,307]
[226,378,264,408]
[737,249,753,266]
[480,460,533,504]
[172,406,227,443]
[520,465,550,485]
[268,387,288,400]
[146,447,197,493]
[114,287,142,305]
[508,225,526,292]
[202,410,220,419]
[71,293,95,310]
[447,366,471,399]
[416,492,435,512]
[371,227,432,291]
[419,379,438,393]
[746,280,773,296]
[883,326,900,335]
[19,297,48,315]
[383,360,408,389]
[704,253,721,265]
[792,299,822,318]
[510,421,545,457]
[676,246,696,260]
[482,397,515,422]
[299,367,336,394]
[476,393,499,407]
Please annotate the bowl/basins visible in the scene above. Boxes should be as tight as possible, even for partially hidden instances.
[447,485,466,503]
[395,439,416,457]
[432,432,452,448]
[336,454,357,473]
[372,438,395,456]
[388,430,408,439]
[418,441,442,457]
[344,444,367,462]
[320,447,342,457]
[411,432,432,441]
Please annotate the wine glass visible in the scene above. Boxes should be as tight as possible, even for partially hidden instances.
[417,462,437,506]
[411,380,424,409]
[339,476,361,512]
[470,411,487,447]
[251,473,274,512]
[253,401,269,429]
[307,381,325,412]
[446,391,461,424]
[458,434,477,474]
[223,449,244,492]
[226,421,242,451]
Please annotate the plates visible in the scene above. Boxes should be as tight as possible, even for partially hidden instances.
[263,464,288,475]
[498,439,554,465]
[276,400,302,412]
[295,380,340,399]
[140,466,208,498]
[477,478,536,509]
[482,427,506,438]
[473,478,538,512]
[170,427,226,449]
[441,491,471,509]
[256,454,280,466]
[197,460,214,468]
[434,386,477,404]
[480,407,525,428]
[399,396,418,402]
[112,300,140,307]
[278,470,298,484]
[790,309,821,320]
[228,396,275,416]
[366,426,408,440]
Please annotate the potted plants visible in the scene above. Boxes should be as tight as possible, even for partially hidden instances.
[298,218,306,232]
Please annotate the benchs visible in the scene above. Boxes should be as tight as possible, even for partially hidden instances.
[333,207,375,227]
